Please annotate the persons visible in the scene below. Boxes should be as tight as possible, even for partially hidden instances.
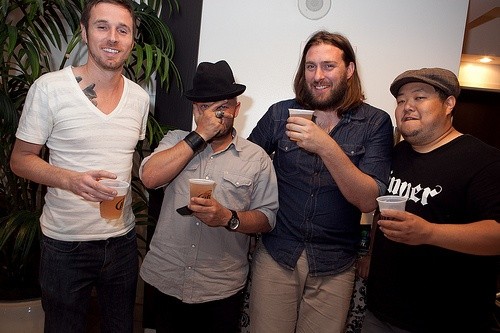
[9,0,150,333]
[246,31,393,333]
[359,68,500,333]
[139,61,280,333]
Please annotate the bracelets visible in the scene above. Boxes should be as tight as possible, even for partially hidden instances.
[183,131,207,156]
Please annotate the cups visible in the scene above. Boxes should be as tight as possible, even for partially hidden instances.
[189,178,215,206]
[288,108,314,141]
[376,195,408,222]
[98,180,130,220]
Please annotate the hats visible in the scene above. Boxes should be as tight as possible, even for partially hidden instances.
[186,60,246,103]
[390,68,461,99]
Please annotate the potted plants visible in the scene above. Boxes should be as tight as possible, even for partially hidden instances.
[0,0,187,333]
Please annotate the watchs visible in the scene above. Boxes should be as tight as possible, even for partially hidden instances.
[224,209,240,232]
